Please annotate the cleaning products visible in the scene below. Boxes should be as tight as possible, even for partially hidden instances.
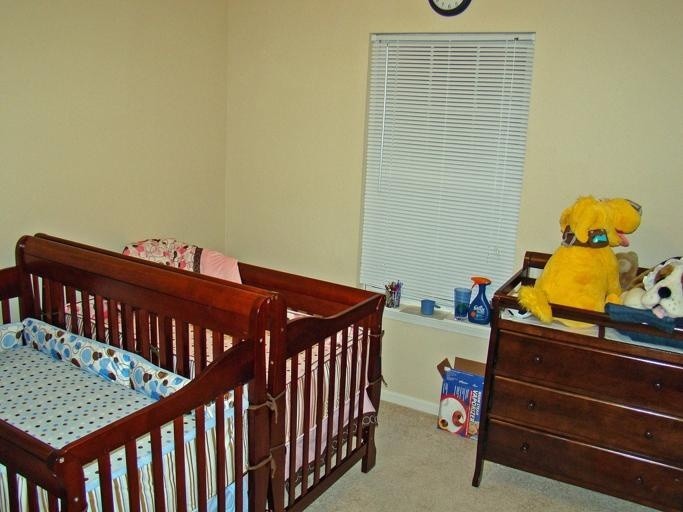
[468,277,490,324]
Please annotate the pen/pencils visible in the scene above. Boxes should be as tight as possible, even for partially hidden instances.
[385,280,403,308]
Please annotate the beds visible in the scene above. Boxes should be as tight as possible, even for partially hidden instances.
[2,231,270,512]
[35,231,385,512]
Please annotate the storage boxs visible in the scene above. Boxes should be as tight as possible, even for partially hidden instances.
[436,356,487,441]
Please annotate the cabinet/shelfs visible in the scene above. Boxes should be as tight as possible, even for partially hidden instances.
[472,251,683,512]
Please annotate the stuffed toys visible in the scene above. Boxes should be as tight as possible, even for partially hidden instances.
[613,251,638,293]
[620,257,683,320]
[517,194,643,330]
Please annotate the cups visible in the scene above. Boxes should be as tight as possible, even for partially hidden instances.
[453,287,472,321]
[385,291,401,309]
[420,299,441,315]
